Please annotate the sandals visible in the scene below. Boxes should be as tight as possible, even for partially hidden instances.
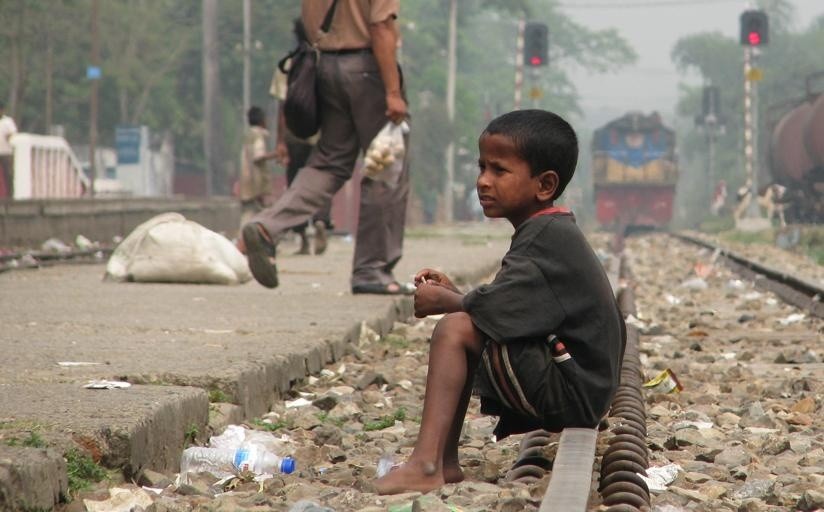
[243,221,277,288]
[350,280,414,295]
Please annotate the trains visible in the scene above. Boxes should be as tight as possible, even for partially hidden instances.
[589,107,679,231]
[755,68,824,224]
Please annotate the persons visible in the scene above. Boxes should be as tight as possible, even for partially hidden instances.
[235,105,279,254]
[373,111,628,493]
[244,1,416,294]
[0,98,17,200]
[710,177,786,226]
[269,16,331,253]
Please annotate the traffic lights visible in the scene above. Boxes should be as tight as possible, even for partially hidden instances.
[741,10,766,45]
[523,20,546,68]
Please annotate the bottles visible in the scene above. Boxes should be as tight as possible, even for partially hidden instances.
[178,443,296,476]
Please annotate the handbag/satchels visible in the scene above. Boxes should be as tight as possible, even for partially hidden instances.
[279,39,318,139]
